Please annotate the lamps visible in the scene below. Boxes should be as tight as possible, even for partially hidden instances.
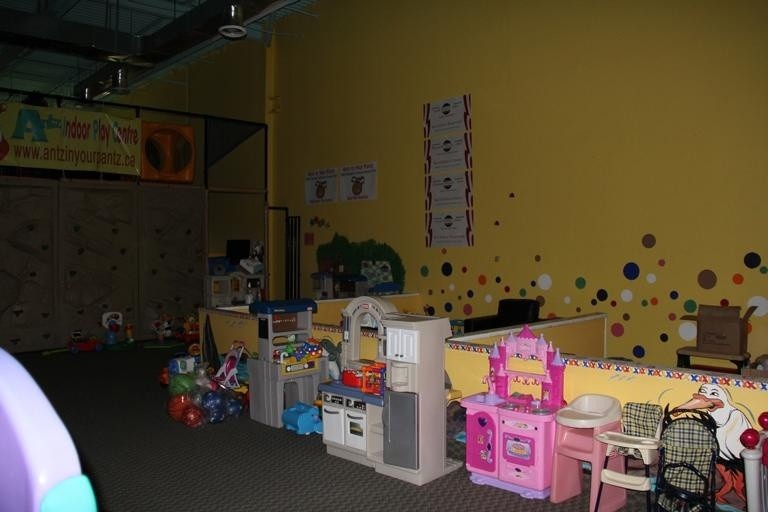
[217,0,248,39]
[110,63,129,95]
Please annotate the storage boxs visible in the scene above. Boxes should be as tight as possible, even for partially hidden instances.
[680,304,758,356]
